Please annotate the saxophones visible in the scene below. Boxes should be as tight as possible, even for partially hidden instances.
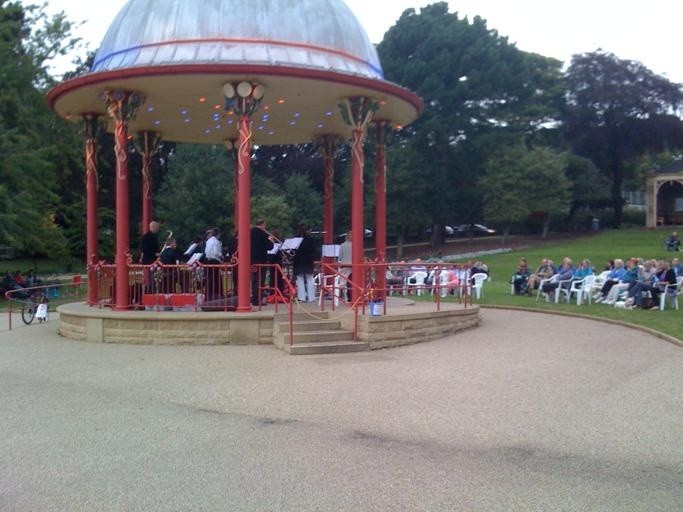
[158,230,173,258]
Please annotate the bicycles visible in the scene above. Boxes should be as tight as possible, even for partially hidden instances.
[20,282,56,324]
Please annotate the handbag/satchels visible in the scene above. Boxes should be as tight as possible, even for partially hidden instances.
[615,301,625,308]
[642,297,658,308]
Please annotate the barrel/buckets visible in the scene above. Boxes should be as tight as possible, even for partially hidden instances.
[369,301,384,316]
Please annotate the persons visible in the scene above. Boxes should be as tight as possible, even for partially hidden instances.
[663,230,681,251]
[137,220,238,300]
[508,257,683,311]
[0,268,60,299]
[249,218,274,307]
[386,256,489,299]
[292,223,318,303]
[266,228,286,295]
[332,231,352,301]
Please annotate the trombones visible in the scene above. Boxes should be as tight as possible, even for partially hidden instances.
[263,228,296,256]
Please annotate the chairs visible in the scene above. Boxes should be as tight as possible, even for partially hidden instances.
[390,266,683,312]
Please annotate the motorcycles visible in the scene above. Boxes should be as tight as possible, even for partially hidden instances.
[2,270,46,302]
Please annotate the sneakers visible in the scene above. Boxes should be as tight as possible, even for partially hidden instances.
[619,292,635,306]
[593,292,614,305]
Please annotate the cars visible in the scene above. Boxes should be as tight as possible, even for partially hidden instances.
[423,224,497,239]
[306,226,374,243]
